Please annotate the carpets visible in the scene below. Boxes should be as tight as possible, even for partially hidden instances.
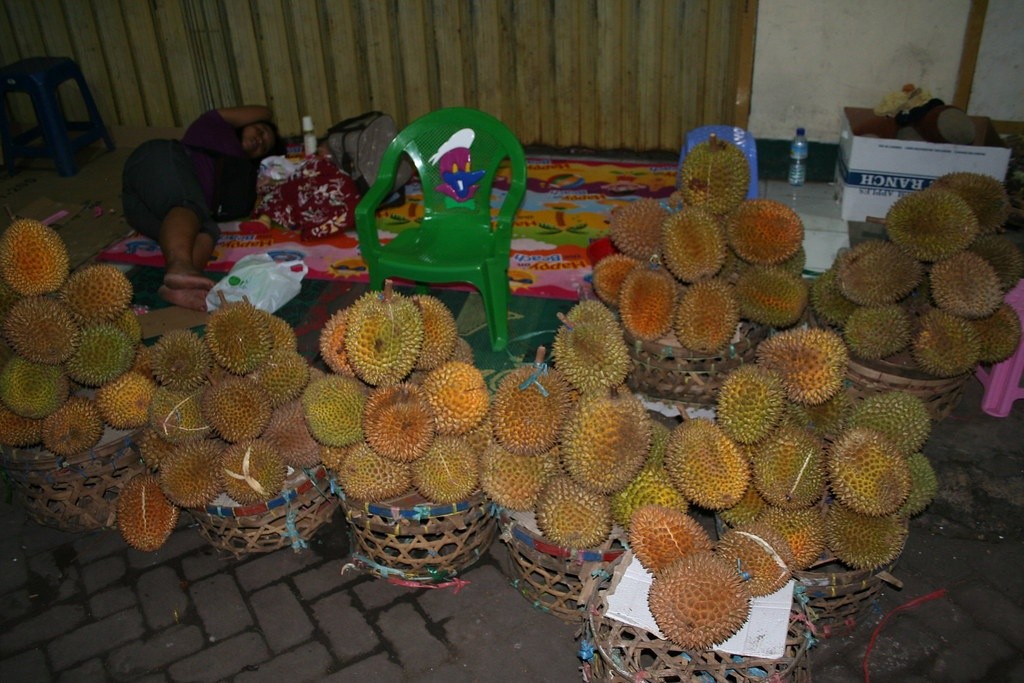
[58,260,576,416]
[94,156,689,302]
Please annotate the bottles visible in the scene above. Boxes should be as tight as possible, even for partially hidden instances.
[788,128,809,186]
[302,116,318,157]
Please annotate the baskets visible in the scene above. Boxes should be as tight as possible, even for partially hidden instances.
[2,294,978,683]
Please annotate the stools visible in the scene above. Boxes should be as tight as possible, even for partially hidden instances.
[0,56,116,176]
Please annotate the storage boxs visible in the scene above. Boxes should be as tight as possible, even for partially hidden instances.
[834,105,1013,223]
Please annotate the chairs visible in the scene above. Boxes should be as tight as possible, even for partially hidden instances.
[666,125,760,222]
[972,278,1024,418]
[353,105,527,353]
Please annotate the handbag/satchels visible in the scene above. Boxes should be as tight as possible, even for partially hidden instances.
[206,252,308,312]
[330,113,411,199]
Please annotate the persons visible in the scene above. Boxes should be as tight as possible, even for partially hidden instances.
[120,102,282,312]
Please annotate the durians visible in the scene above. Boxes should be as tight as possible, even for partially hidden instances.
[0,133,1022,651]
